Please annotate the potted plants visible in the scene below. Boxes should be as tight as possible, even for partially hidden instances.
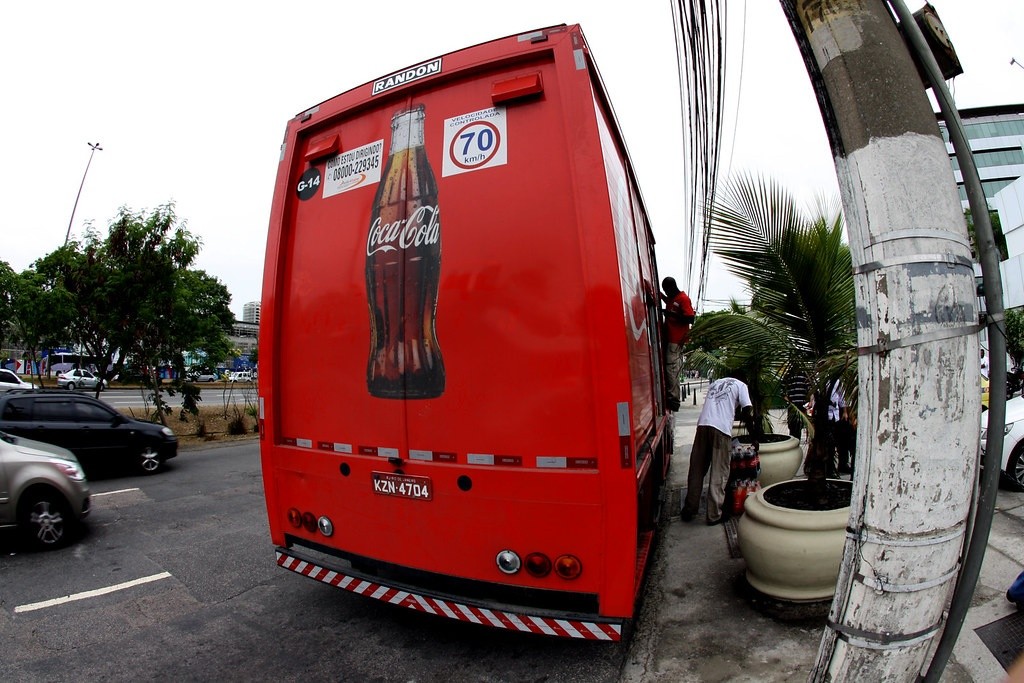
[673,171,858,603]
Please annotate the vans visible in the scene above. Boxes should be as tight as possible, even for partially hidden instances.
[227,372,252,382]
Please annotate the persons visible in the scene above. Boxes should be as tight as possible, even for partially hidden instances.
[781,361,857,479]
[660,277,695,412]
[680,370,760,526]
[683,367,714,385]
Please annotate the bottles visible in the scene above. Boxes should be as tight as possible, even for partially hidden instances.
[365,103,444,399]
[731,443,761,511]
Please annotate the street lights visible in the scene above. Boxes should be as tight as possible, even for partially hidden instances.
[61,140,104,246]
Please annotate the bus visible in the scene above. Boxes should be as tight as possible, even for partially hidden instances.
[45,352,114,376]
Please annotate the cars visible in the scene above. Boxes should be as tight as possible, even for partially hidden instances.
[58,368,110,391]
[0,368,41,404]
[980,390,1024,488]
[0,430,94,550]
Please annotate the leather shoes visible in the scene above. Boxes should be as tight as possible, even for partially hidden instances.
[829,470,841,479]
[839,466,853,474]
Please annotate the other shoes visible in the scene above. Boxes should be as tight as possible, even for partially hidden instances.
[681,514,696,522]
[665,401,683,411]
[706,518,724,526]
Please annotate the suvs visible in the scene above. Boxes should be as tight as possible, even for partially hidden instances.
[0,388,178,474]
[185,368,216,382]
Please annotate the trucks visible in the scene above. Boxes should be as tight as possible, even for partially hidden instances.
[256,22,675,642]
